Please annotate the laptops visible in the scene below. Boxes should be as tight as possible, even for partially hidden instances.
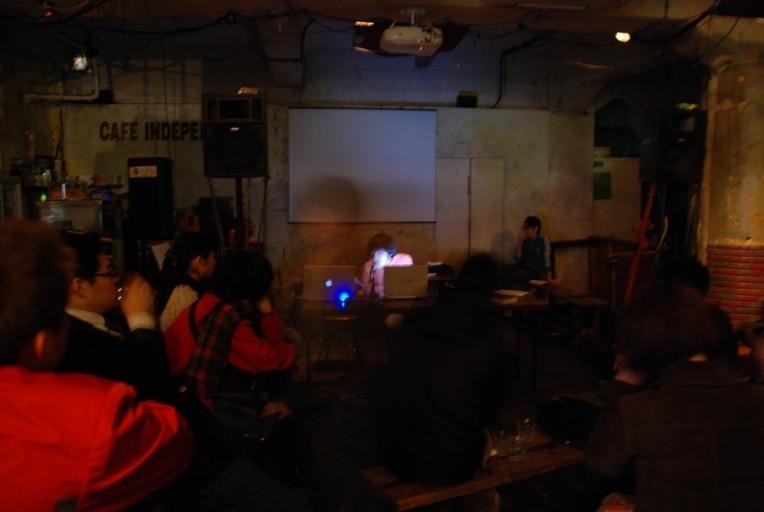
[303,264,355,303]
[379,265,429,300]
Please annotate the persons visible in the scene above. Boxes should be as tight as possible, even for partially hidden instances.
[0,219,295,512]
[509,215,547,283]
[535,267,763,511]
[366,255,507,481]
[368,233,395,259]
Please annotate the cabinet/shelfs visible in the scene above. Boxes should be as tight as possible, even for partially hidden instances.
[32,188,129,283]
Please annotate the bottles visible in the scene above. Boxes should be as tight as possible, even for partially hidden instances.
[25,168,120,200]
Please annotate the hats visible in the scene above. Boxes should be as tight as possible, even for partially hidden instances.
[521,216,541,229]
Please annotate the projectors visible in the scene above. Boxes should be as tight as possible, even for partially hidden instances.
[349,18,444,58]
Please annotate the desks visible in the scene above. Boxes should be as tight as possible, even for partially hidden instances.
[318,286,611,402]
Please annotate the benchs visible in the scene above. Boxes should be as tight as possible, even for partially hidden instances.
[352,416,596,512]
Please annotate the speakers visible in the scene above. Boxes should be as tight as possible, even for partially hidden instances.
[641,107,708,183]
[200,91,270,178]
